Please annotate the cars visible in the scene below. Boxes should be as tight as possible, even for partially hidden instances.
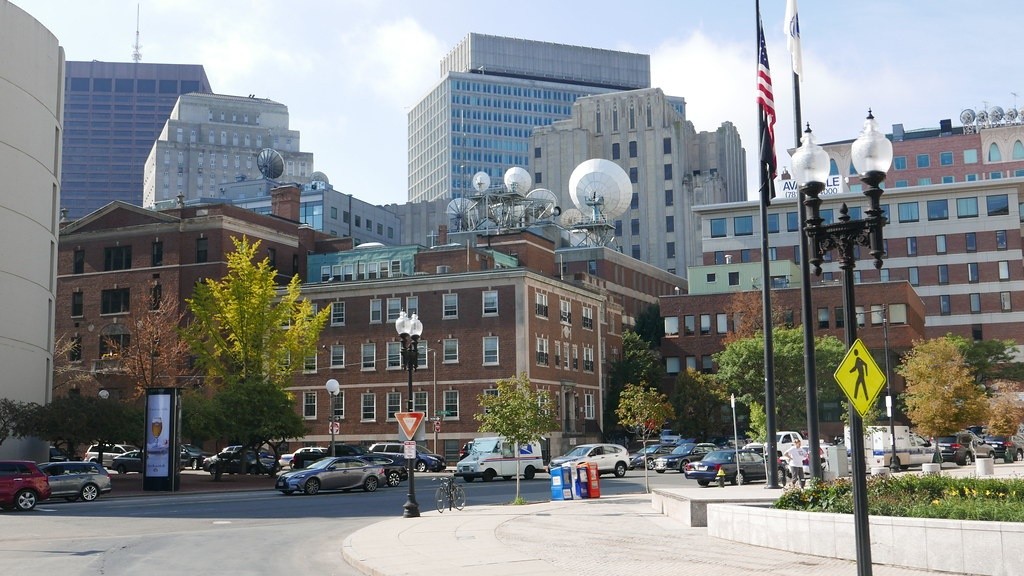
[275,456,387,496]
[653,441,721,474]
[355,453,408,488]
[925,429,996,466]
[49,445,76,462]
[684,447,789,486]
[628,437,699,470]
[658,429,684,445]
[725,429,846,479]
[967,425,1024,462]
[111,449,142,475]
[180,442,215,470]
[201,441,382,478]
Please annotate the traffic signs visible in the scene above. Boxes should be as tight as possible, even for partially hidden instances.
[430,416,441,421]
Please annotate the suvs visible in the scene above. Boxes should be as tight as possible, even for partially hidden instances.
[0,458,52,513]
[37,459,113,502]
[547,443,630,479]
[82,443,140,470]
[367,441,448,473]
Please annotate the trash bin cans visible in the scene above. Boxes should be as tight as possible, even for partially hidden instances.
[550,466,574,500]
[575,462,600,500]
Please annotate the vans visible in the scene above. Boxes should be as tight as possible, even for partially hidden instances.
[453,434,546,483]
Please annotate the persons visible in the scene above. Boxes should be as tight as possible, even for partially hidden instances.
[784,439,808,490]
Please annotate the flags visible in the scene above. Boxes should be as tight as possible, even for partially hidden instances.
[756,23,778,179]
[783,0,803,83]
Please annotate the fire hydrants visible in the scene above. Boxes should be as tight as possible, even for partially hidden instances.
[714,466,727,487]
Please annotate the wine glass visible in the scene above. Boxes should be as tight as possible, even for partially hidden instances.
[152,417,162,448]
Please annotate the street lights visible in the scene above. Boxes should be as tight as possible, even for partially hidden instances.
[417,345,438,454]
[395,307,424,519]
[786,106,895,576]
[97,389,110,467]
[325,378,340,457]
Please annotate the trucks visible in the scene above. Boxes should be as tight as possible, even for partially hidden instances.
[841,422,936,474]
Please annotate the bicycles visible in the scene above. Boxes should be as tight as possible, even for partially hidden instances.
[431,470,467,513]
